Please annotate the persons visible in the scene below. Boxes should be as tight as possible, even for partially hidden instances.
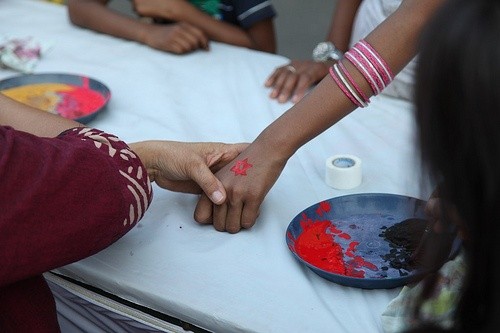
[68,0,278,54]
[0,94,251,332]
[194,0,448,233]
[413,0,500,333]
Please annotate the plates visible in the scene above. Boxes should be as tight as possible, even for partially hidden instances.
[285,193,463,290]
[0,73,112,125]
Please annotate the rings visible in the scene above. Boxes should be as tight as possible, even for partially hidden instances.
[285,64,297,75]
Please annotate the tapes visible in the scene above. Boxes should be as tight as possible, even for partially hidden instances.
[321,153,366,191]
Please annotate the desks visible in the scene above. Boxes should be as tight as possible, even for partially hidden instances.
[0,0,437,333]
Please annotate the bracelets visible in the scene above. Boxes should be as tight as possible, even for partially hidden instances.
[329,39,394,109]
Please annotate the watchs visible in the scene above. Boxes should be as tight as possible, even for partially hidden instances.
[311,41,343,62]
